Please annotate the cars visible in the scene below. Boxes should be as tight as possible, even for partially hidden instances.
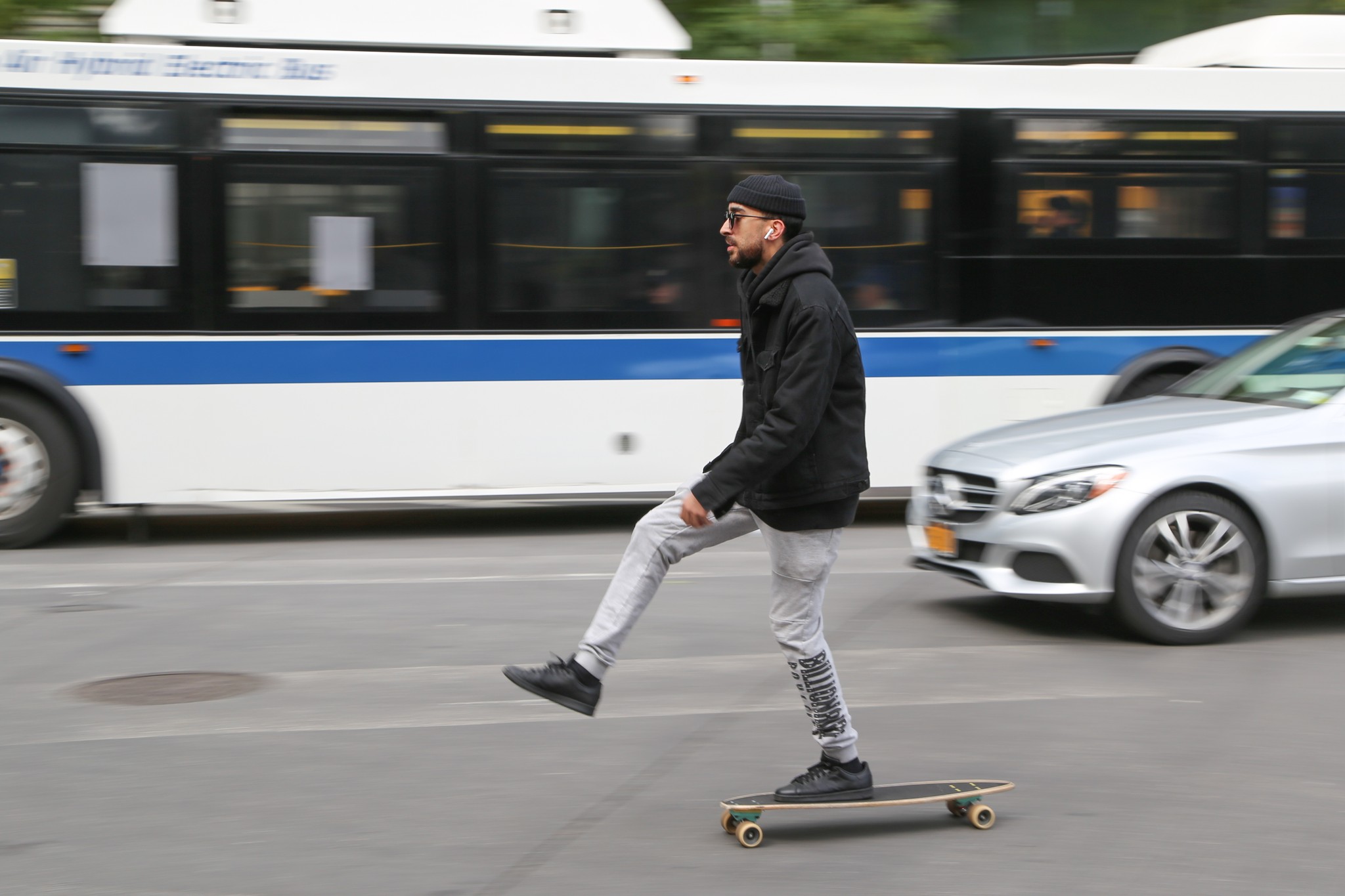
[904,304,1345,646]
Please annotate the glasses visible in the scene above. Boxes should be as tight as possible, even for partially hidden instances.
[724,210,777,230]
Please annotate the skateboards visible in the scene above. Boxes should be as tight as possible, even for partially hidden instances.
[718,778,1016,847]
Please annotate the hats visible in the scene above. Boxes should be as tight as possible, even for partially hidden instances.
[727,175,807,219]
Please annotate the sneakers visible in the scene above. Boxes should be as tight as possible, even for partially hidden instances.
[500,651,602,717]
[774,761,874,803]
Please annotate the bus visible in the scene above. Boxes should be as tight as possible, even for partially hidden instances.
[0,3,1345,547]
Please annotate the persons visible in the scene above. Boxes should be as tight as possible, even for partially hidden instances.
[500,174,874,802]
